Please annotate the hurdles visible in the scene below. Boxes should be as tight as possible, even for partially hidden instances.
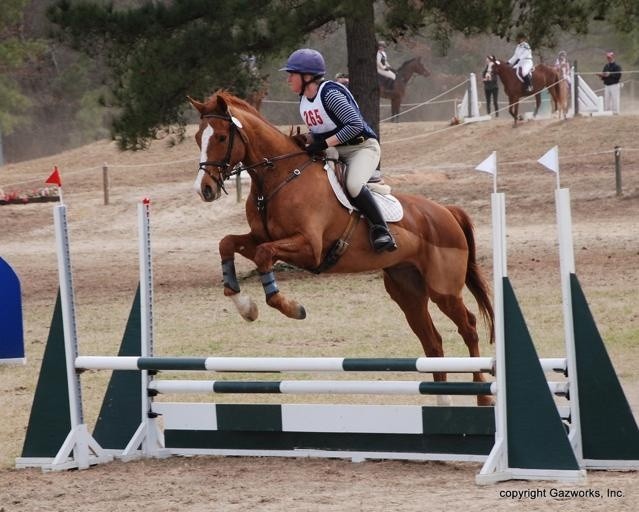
[15,189,639,486]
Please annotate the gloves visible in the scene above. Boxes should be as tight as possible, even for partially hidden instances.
[308,140,328,154]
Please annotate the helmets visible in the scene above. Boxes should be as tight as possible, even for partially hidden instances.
[278,49,326,75]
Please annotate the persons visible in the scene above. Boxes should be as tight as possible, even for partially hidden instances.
[506,32,534,94]
[481,58,499,120]
[556,51,572,92]
[596,51,622,114]
[376,40,397,94]
[281,48,398,252]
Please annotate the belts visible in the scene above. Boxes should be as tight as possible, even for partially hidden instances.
[349,134,369,144]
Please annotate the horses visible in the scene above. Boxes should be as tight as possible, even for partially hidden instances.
[334,56,431,123]
[184,88,497,408]
[483,53,570,128]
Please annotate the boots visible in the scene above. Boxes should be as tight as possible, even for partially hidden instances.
[352,184,397,252]
[523,73,533,92]
